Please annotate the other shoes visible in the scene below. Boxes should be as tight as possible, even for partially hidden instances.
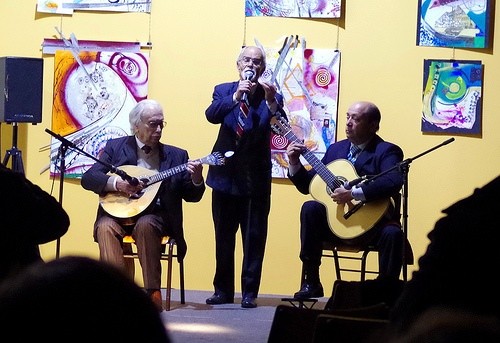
[148,290,163,311]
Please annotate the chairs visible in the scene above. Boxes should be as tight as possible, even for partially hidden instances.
[293,170,409,308]
[124,234,186,311]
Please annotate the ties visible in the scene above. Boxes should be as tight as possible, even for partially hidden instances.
[347,145,361,164]
[235,101,250,145]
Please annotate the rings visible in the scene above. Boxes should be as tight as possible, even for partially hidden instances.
[129,194,131,197]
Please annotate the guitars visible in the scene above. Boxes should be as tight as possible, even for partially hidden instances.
[268,111,395,247]
[99,150,225,219]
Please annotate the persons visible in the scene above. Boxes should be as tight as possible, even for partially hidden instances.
[0,256,169,343]
[205,46,289,309]
[286,102,414,299]
[81,100,205,313]
[0,163,70,285]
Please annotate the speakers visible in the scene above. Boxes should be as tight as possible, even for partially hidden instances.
[0,56,44,125]
[268,306,392,343]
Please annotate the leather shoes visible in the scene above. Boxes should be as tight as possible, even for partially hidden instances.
[294,281,324,298]
[206,293,234,305]
[241,296,257,308]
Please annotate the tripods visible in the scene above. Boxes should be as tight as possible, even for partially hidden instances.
[2,122,25,176]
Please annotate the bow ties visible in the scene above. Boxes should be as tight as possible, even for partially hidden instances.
[142,146,158,159]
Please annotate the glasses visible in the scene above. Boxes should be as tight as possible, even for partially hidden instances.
[140,118,166,128]
[238,56,264,66]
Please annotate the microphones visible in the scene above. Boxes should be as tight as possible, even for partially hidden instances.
[109,165,139,186]
[344,175,366,190]
[240,70,254,102]
